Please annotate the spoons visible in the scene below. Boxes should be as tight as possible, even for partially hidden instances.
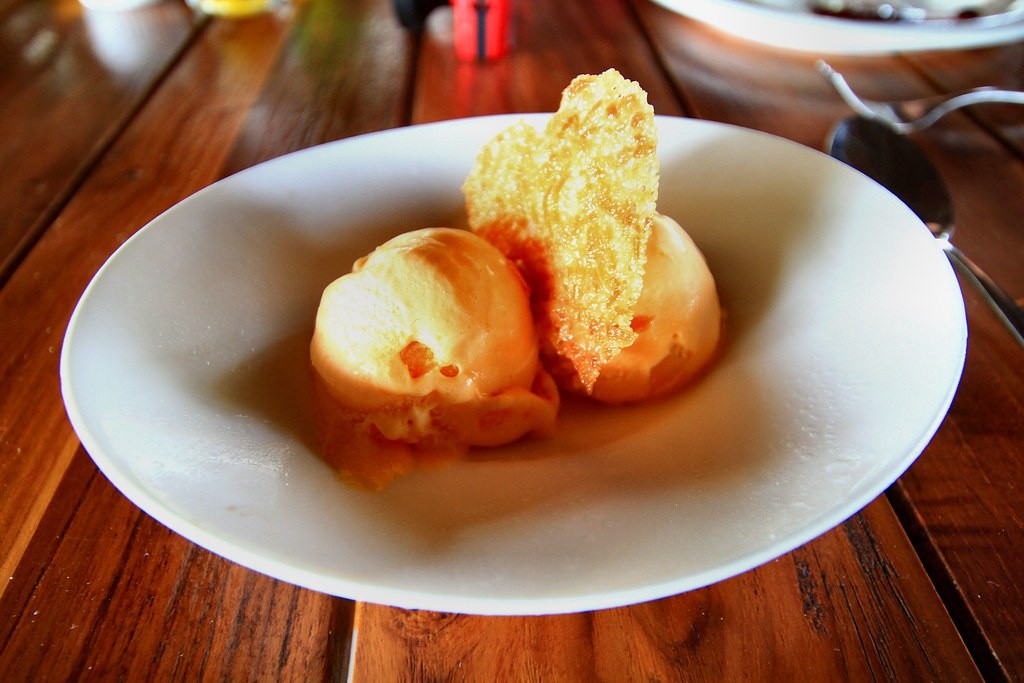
[827,114,1024,348]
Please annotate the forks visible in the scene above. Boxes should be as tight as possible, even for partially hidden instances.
[818,58,1023,134]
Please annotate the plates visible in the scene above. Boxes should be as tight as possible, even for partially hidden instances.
[61,112,969,618]
